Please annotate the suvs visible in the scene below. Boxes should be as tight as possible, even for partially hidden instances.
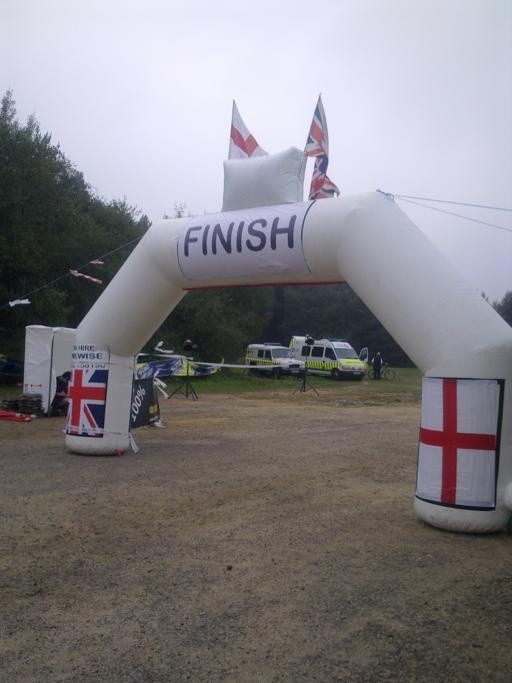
[245,342,305,378]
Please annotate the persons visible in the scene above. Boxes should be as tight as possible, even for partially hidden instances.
[371,351,383,379]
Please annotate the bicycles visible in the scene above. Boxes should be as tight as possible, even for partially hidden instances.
[367,362,396,380]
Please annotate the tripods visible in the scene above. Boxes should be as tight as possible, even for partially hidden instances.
[168,352,198,401]
[293,344,320,397]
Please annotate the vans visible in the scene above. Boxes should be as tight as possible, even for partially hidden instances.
[288,335,369,380]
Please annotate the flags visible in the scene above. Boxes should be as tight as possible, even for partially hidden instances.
[305,96,342,200]
[228,100,270,159]
